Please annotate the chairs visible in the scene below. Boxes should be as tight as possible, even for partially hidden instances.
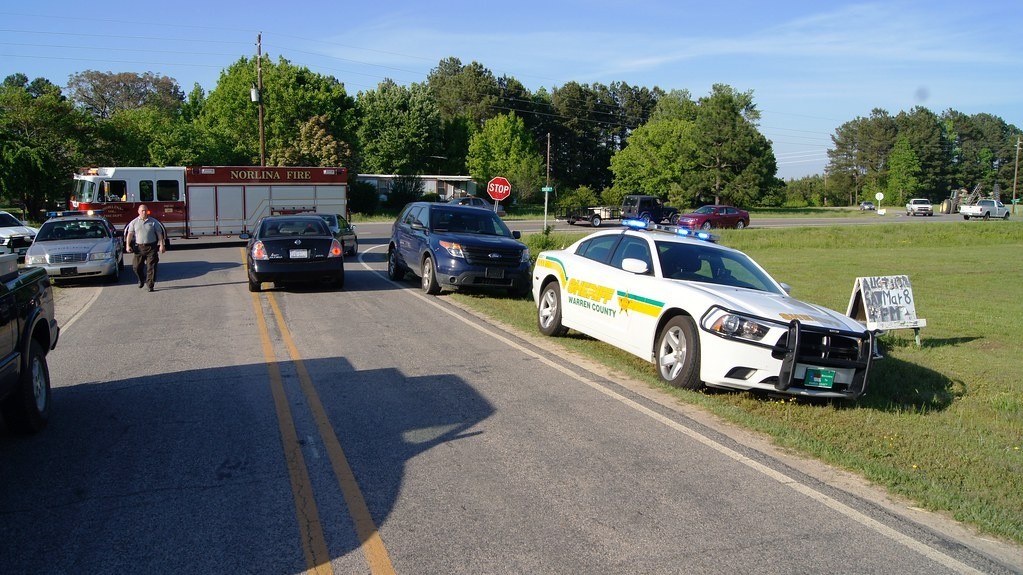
[620,241,649,272]
[54,227,65,237]
[89,225,98,234]
[670,254,702,279]
[303,227,317,235]
[266,229,278,236]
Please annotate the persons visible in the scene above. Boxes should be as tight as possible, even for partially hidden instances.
[126,204,165,292]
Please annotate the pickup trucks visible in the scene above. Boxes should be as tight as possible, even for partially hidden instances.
[904,198,933,216]
[1,253,59,428]
[958,198,1010,220]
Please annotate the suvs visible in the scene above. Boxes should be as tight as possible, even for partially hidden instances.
[0,209,38,258]
[619,193,681,225]
[386,202,530,295]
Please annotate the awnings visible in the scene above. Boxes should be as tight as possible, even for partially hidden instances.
[440,178,471,181]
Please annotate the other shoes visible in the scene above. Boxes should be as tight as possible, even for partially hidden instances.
[149,280,155,292]
[138,278,144,288]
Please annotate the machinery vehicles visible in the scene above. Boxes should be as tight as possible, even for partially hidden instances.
[938,189,970,213]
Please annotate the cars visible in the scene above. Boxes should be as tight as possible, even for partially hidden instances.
[678,204,750,230]
[24,209,124,285]
[448,196,505,217]
[239,214,345,291]
[859,201,875,211]
[313,214,357,255]
[532,218,873,398]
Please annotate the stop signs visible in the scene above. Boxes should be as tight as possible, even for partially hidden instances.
[486,176,511,201]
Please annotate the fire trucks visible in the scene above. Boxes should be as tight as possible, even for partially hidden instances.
[67,164,351,253]
